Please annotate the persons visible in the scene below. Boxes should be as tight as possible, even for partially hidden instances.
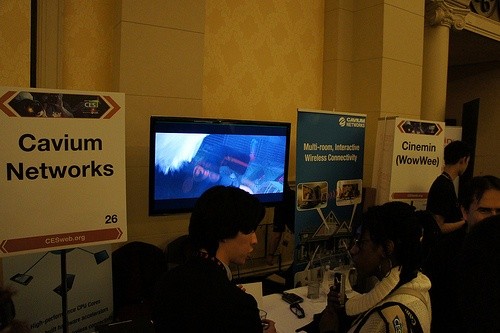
[138,185,277,333]
[314,140,500,333]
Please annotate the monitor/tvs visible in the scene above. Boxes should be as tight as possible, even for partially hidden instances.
[148,115,292,217]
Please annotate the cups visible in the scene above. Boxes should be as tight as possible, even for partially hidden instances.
[259,309,267,327]
[307,282,320,299]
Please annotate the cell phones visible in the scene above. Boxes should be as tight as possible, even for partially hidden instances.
[282,293,304,304]
[333,271,346,306]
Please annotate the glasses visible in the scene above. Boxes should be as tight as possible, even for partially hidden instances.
[355,236,373,246]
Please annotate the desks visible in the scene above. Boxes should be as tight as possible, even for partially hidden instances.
[263,285,359,333]
